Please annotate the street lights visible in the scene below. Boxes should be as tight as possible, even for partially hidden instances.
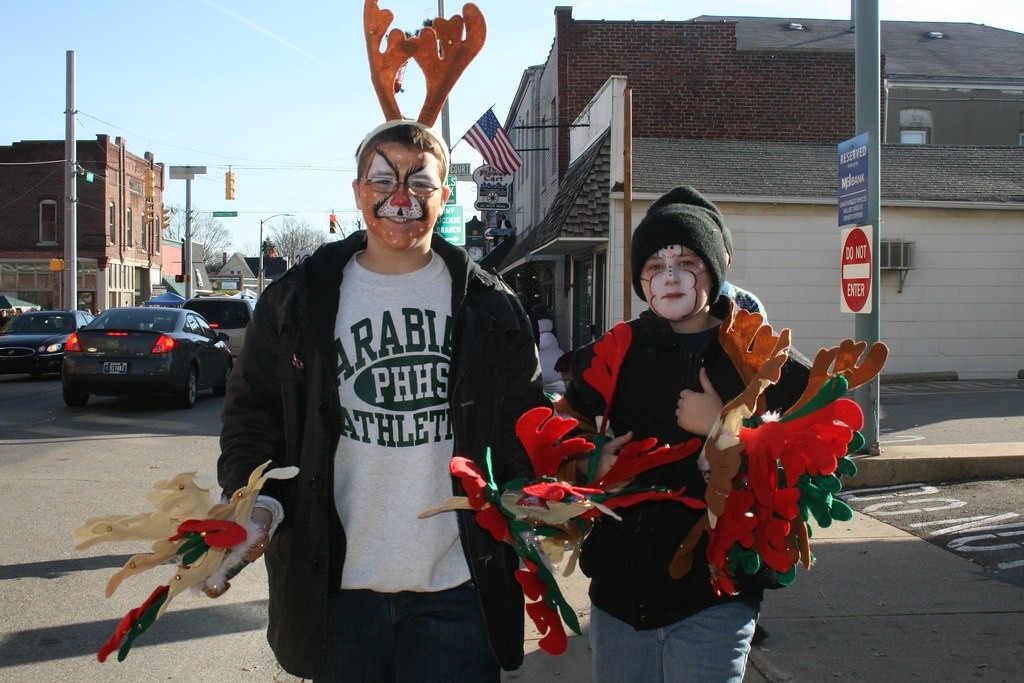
[259,213,297,294]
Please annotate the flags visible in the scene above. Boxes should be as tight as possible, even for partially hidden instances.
[462,109,524,175]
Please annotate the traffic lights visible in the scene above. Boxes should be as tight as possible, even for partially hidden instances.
[145,201,154,225]
[161,208,170,229]
[329,215,337,232]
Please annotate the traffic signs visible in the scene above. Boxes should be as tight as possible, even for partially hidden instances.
[433,205,465,245]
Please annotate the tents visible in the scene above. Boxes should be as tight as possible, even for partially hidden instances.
[145,292,186,309]
[0,294,41,313]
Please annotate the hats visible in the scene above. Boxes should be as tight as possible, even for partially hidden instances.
[631,185,734,304]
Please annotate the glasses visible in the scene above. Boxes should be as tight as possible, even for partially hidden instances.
[358,177,443,198]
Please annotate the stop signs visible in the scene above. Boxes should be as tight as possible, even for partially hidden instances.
[838,225,875,316]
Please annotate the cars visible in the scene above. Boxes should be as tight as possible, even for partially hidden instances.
[61,307,234,408]
[179,294,258,356]
[0,310,96,374]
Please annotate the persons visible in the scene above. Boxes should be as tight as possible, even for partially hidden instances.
[84,308,100,318]
[0,306,18,327]
[215,118,588,683]
[556,186,812,683]
